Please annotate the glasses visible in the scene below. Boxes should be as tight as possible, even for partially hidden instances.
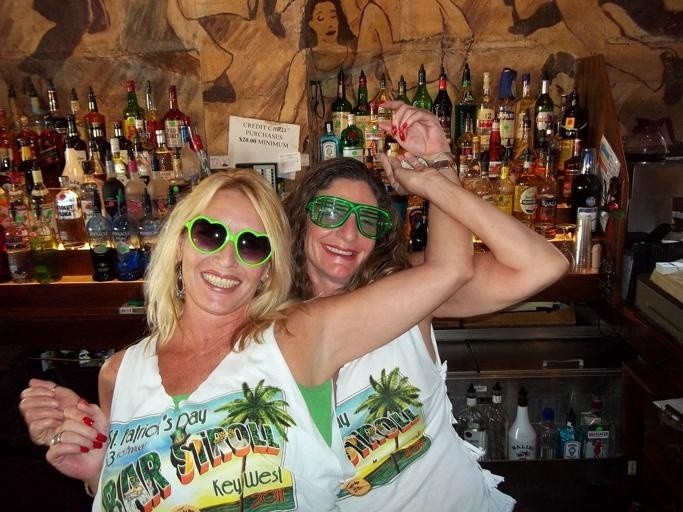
[302,192,395,244]
[178,212,283,271]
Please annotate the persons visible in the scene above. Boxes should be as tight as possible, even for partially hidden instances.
[277,1,357,123]
[45,98,474,512]
[17,156,519,512]
[541,51,578,111]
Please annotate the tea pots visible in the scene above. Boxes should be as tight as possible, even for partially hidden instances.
[620,114,677,170]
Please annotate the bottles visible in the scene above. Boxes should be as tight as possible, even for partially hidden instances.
[455,380,615,463]
[1,73,209,282]
[318,62,606,274]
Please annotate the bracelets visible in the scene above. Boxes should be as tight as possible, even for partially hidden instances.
[432,160,456,171]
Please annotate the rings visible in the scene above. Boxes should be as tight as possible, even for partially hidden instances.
[53,433,63,443]
[49,383,58,391]
